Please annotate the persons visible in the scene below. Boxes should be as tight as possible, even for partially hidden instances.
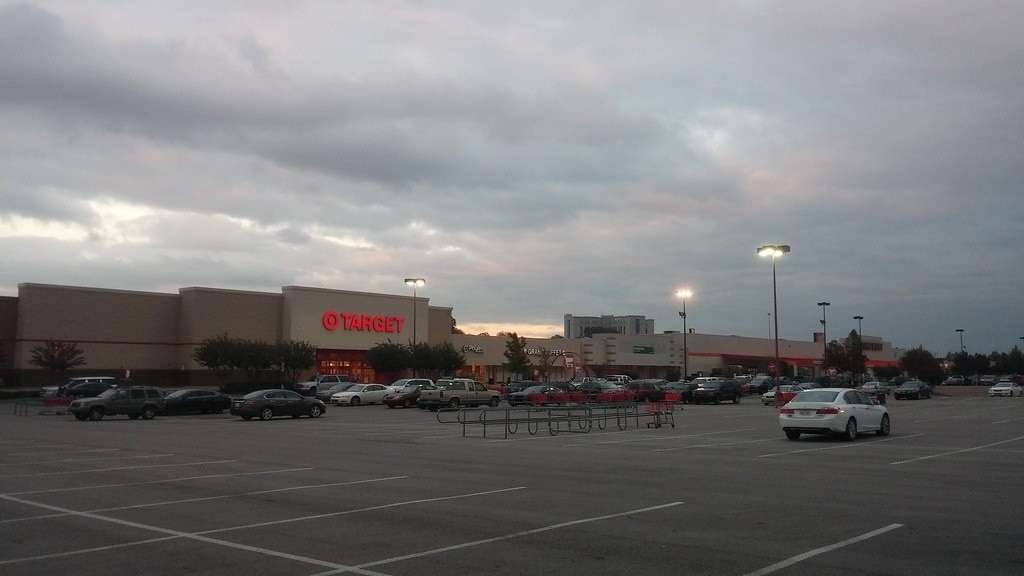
[507,378,511,386]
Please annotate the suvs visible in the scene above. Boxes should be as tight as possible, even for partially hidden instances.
[293,374,351,396]
[419,377,503,411]
[69,387,166,422]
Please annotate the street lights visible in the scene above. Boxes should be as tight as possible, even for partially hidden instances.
[676,290,693,379]
[817,302,833,376]
[404,278,427,379]
[956,328,965,354]
[756,245,791,405]
[852,315,867,344]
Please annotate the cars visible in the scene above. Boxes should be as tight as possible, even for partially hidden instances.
[894,381,932,400]
[230,388,327,420]
[734,375,821,396]
[779,388,891,441]
[383,385,437,408]
[390,378,435,393]
[508,386,563,406]
[1000,374,1024,384]
[980,374,1000,386]
[941,375,967,386]
[315,383,358,405]
[860,382,891,394]
[761,385,804,406]
[159,389,232,416]
[692,379,743,405]
[506,374,691,404]
[41,376,119,401]
[989,381,1023,398]
[331,384,396,406]
